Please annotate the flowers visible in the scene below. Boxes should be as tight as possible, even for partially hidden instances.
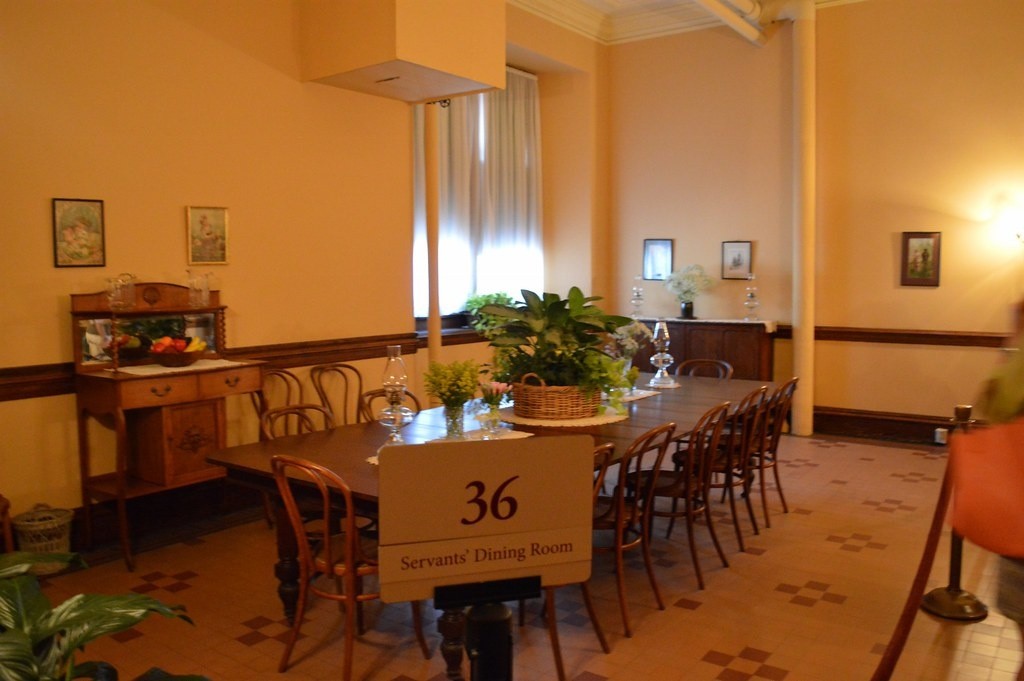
[425,288,655,411]
[663,262,715,299]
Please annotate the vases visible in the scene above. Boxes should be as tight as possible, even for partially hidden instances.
[618,358,633,394]
[483,403,505,436]
[445,404,463,441]
[680,301,693,317]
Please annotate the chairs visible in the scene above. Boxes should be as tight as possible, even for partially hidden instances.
[517,358,800,681]
[249,362,434,680]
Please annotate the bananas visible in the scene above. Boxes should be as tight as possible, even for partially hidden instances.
[184,336,207,352]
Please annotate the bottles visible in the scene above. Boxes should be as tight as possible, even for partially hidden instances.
[376,345,413,454]
[645,321,682,389]
[629,273,645,320]
[743,273,760,321]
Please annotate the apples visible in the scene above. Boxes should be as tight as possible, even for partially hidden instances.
[152,336,186,353]
[117,334,141,349]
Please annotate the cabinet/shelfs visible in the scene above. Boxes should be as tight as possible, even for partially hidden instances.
[69,278,269,571]
[612,316,777,435]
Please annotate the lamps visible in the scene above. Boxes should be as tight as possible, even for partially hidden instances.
[743,292,759,321]
[378,344,414,455]
[630,286,646,319]
[648,322,680,388]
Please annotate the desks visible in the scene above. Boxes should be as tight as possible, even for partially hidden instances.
[203,372,786,681]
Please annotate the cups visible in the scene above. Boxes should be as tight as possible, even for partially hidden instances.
[103,273,136,312]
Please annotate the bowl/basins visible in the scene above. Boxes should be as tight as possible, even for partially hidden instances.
[152,350,201,368]
[953,405,972,421]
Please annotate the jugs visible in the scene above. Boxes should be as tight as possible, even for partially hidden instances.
[185,268,215,308]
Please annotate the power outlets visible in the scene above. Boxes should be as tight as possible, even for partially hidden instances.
[935,427,947,444]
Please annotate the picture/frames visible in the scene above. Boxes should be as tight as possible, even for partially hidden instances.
[721,240,752,280]
[643,238,673,281]
[899,231,942,287]
[52,197,106,268]
[184,203,229,267]
